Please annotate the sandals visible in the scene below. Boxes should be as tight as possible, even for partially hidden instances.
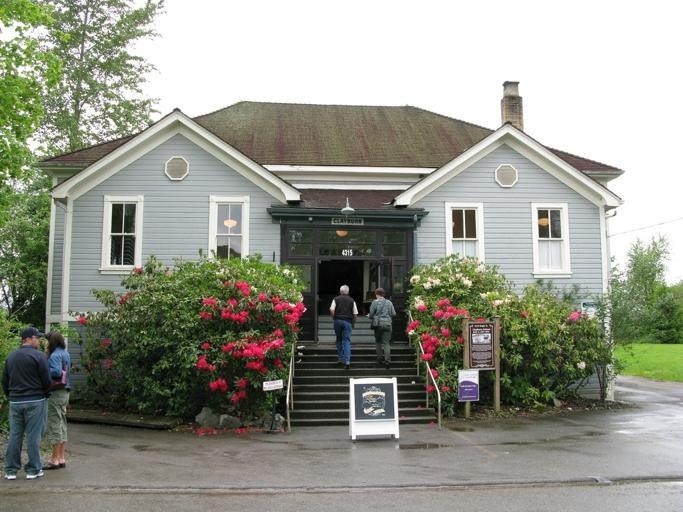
[42,462,65,470]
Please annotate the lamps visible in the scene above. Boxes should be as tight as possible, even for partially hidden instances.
[340,197,355,215]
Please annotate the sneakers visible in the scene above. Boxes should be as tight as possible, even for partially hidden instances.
[26,470,44,480]
[4,474,17,480]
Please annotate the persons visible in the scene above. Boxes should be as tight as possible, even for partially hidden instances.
[329,284,358,369]
[41,331,71,471]
[365,288,395,369]
[2,327,49,481]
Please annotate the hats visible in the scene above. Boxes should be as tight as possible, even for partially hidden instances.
[22,327,45,339]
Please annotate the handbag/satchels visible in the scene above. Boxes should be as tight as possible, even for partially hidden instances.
[51,370,67,386]
[371,316,378,330]
